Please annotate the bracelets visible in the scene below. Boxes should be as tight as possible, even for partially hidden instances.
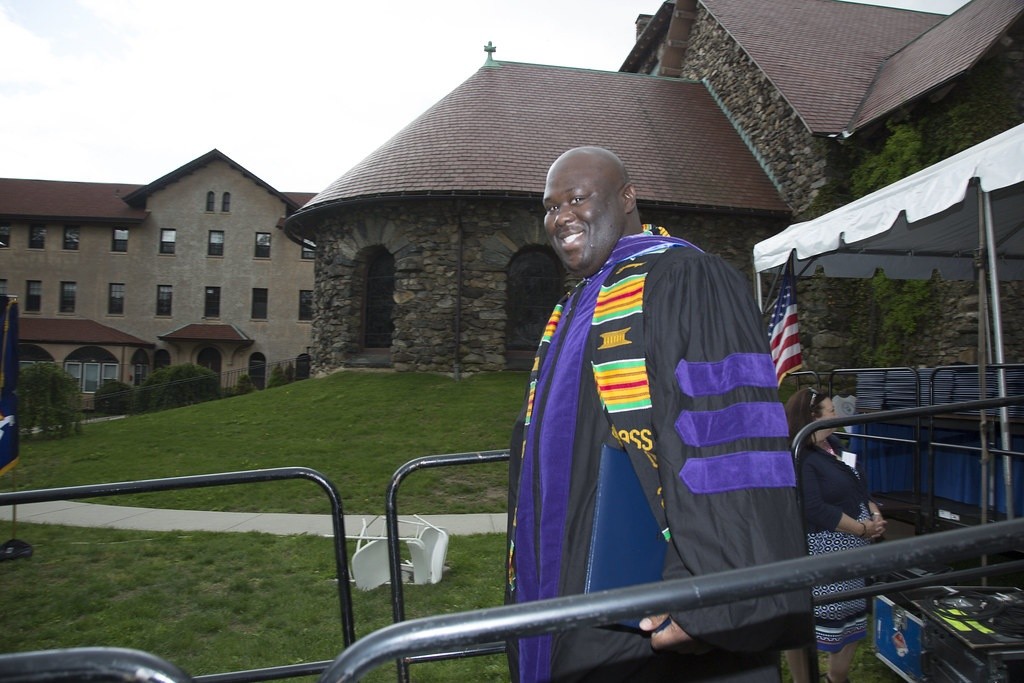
[870,512,881,516]
[857,522,866,538]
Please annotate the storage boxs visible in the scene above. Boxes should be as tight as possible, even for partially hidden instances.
[875,593,924,683]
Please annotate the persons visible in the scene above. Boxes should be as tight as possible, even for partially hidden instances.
[786,388,887,683]
[504,147,812,683]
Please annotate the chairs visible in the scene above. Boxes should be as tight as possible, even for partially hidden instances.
[322,513,451,591]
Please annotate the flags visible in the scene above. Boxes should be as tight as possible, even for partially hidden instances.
[0,300,19,477]
[767,278,803,388]
